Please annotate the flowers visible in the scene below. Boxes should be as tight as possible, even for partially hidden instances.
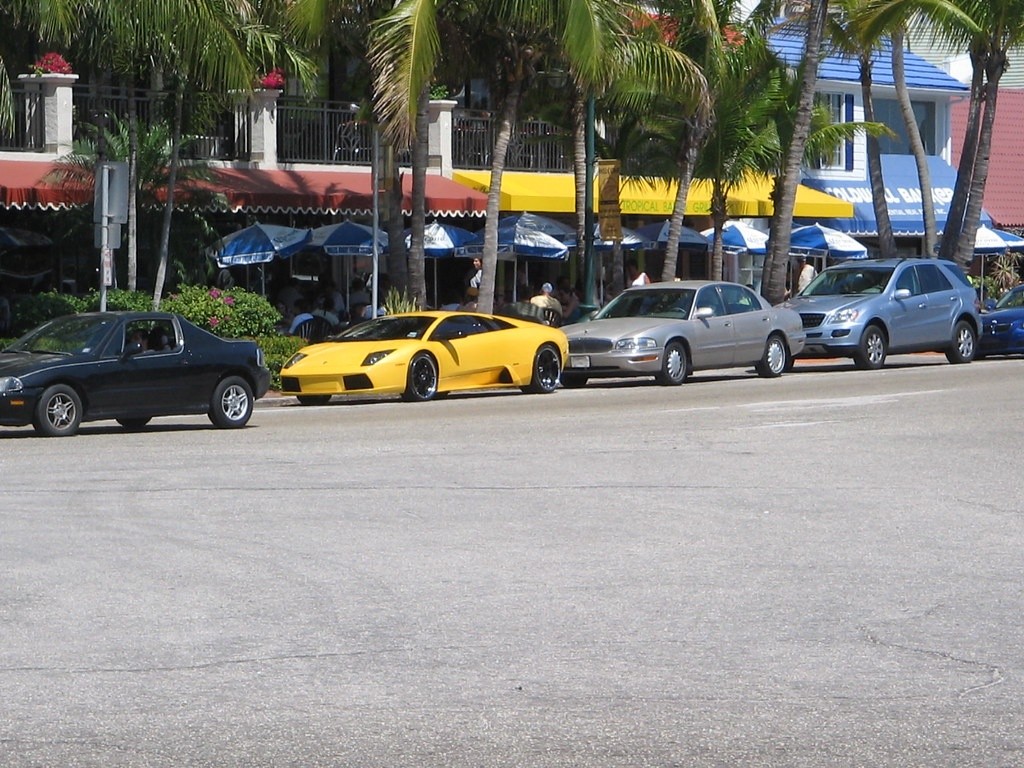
[28,51,73,75]
[250,66,284,89]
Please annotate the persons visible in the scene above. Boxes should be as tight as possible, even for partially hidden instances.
[130,329,149,354]
[465,257,496,304]
[623,259,651,288]
[532,277,581,324]
[274,274,387,329]
[791,254,817,292]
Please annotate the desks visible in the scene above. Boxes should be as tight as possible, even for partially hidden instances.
[194,135,229,159]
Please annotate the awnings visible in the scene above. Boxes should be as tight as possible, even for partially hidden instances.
[789,152,991,235]
[0,159,489,218]
[452,170,854,218]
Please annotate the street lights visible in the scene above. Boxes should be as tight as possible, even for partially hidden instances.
[348,103,379,320]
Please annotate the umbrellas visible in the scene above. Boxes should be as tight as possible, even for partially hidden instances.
[204,222,312,297]
[765,222,868,279]
[453,211,657,308]
[934,224,1024,301]
[301,220,390,313]
[636,220,707,251]
[397,221,474,309]
[699,220,769,255]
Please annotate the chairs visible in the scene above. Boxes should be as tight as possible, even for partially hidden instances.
[707,289,718,316]
[148,326,172,352]
[293,314,331,342]
[334,120,367,162]
[853,278,871,291]
[728,304,750,311]
[543,307,561,329]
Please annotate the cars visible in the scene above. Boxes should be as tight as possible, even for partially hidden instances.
[0,310,271,429]
[979,284,1024,360]
[554,280,806,386]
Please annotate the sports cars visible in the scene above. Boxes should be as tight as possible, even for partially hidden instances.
[278,309,570,406]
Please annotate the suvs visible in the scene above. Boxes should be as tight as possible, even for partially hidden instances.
[772,254,984,370]
